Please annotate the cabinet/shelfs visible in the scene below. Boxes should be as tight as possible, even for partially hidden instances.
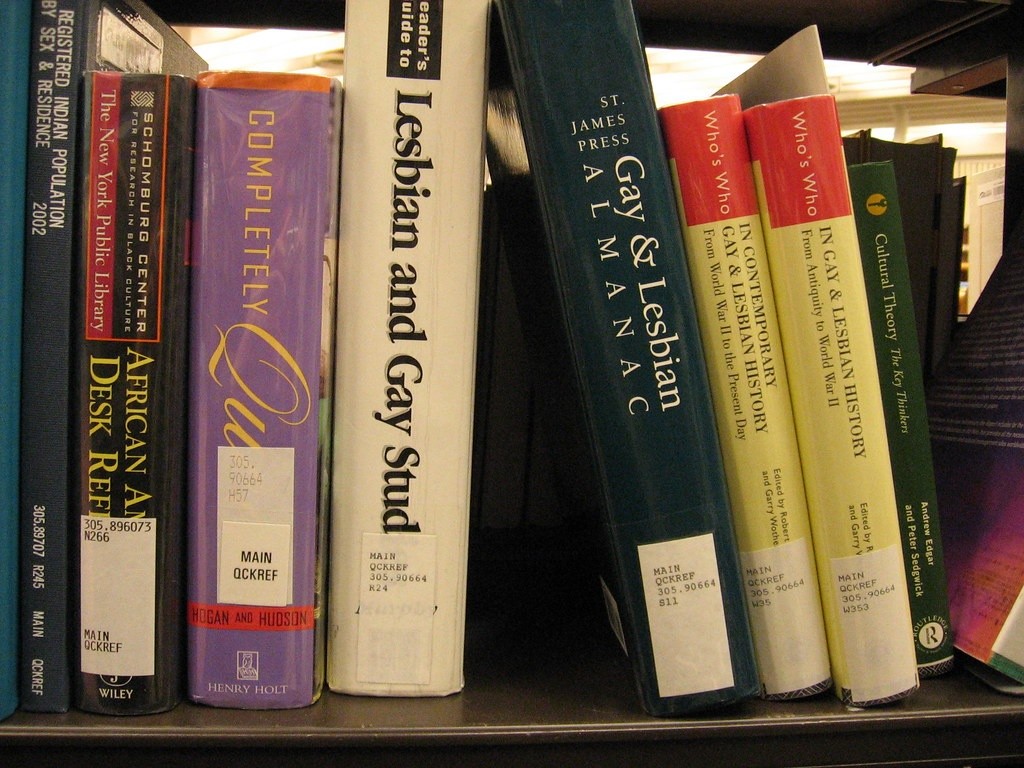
[0,0,1024,768]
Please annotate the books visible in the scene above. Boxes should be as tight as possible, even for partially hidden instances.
[657,92,834,700]
[1,0,1024,719]
